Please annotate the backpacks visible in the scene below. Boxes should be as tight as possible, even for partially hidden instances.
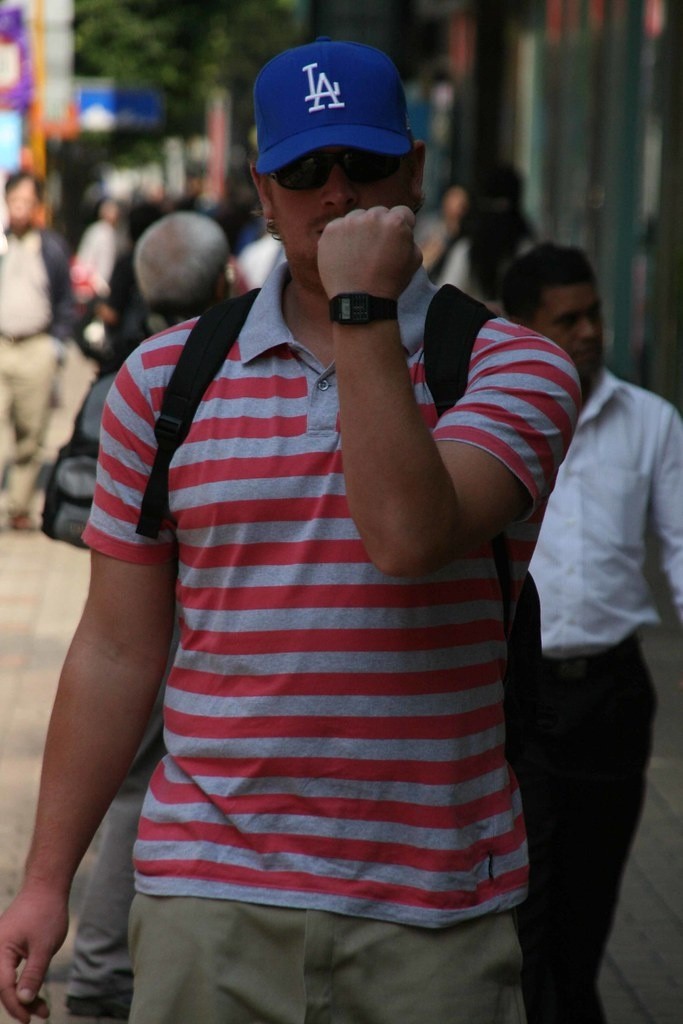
[137,285,545,761]
[40,334,147,550]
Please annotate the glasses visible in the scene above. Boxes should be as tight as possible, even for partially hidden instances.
[270,150,401,190]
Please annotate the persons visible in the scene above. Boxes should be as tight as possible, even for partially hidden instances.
[0,170,534,530]
[507,240,681,1023]
[1,42,581,1024]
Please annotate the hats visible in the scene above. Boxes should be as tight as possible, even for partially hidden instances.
[255,37,413,175]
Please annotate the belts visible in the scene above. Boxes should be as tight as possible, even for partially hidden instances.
[0,331,44,345]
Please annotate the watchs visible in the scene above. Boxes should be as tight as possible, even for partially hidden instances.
[330,294,398,325]
